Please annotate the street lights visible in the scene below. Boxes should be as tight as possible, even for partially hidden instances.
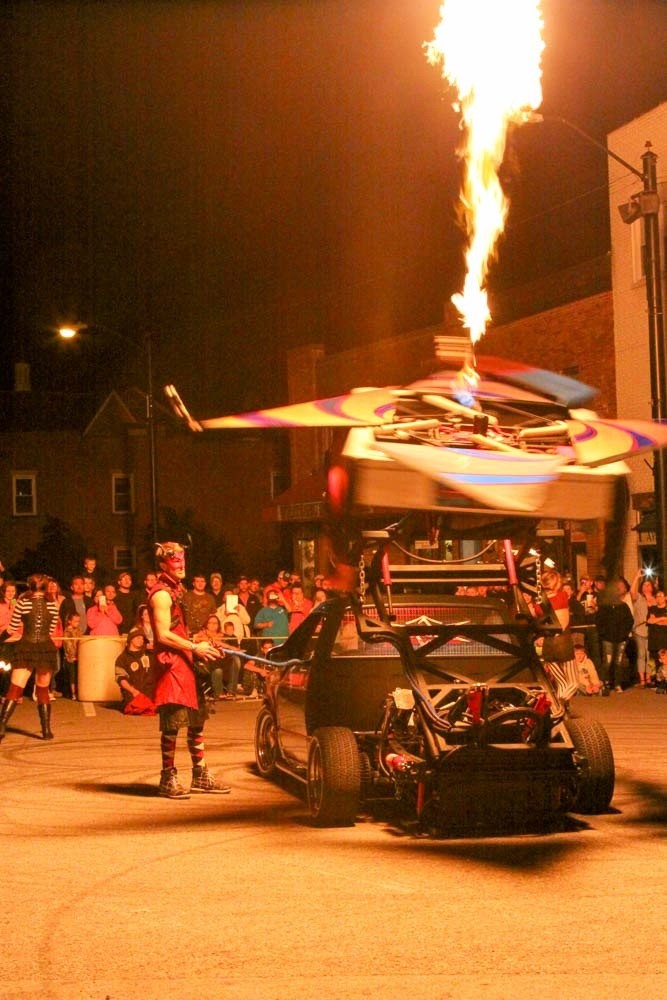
[57,322,160,575]
[509,107,667,596]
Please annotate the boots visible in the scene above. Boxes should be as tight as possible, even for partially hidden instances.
[38,704,53,738]
[190,765,232,794]
[0,699,17,737]
[158,766,191,798]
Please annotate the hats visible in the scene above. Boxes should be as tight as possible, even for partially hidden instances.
[128,627,145,642]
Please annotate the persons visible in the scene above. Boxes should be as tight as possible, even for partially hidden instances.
[0,574,59,741]
[524,571,667,697]
[0,558,326,708]
[147,542,231,799]
[457,586,508,597]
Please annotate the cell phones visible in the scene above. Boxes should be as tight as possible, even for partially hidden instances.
[98,596,106,606]
[269,595,278,599]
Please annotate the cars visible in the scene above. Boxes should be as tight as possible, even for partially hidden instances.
[252,594,616,830]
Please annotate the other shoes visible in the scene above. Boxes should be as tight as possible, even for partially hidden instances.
[640,680,654,689]
[615,685,623,693]
